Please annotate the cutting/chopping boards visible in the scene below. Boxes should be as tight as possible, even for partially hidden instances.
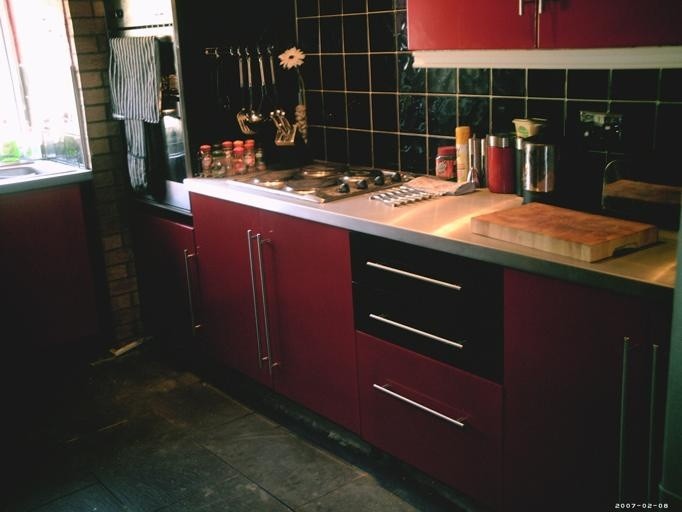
[605,179,681,226]
[470,201,659,264]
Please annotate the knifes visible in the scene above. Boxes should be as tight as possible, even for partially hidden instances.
[269,109,293,137]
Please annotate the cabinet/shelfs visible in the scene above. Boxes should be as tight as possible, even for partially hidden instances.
[132,213,196,358]
[0,186,97,373]
[505,271,673,512]
[406,0,682,70]
[187,190,360,436]
[344,226,507,511]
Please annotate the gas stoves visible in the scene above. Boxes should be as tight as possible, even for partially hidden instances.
[230,159,419,204]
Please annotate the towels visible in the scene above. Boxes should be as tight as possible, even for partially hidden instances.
[110,35,161,187]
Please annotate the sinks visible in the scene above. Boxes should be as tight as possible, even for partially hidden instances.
[0,166,41,178]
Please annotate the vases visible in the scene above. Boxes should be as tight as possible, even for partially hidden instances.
[296,105,308,143]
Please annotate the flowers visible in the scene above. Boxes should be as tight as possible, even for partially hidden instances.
[279,48,305,105]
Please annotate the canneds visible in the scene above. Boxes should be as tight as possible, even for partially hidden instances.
[435,146,457,180]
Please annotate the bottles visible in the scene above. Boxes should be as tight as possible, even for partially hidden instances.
[199,140,266,178]
[455,126,556,205]
